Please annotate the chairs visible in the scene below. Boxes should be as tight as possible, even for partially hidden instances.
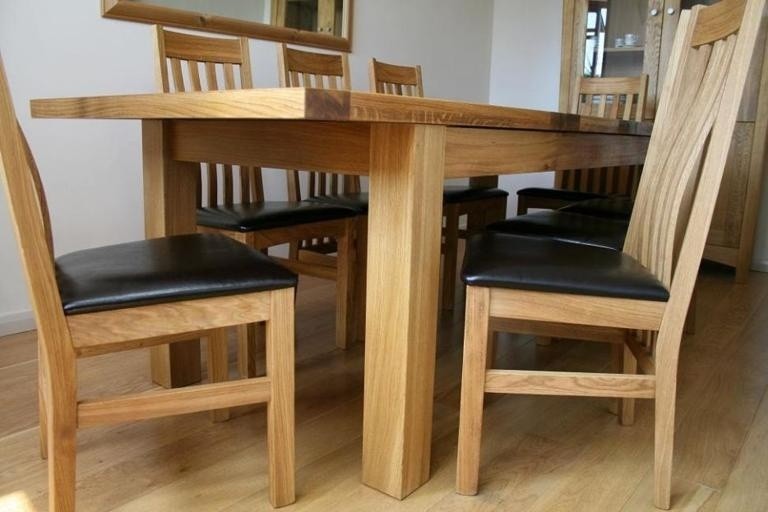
[452,1,768,505]
[149,26,509,344]
[487,1,694,437]
[1,61,301,507]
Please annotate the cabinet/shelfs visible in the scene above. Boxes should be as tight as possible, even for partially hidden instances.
[554,0,768,282]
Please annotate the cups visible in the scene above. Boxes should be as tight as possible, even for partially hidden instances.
[615,37,626,48]
[626,34,639,47]
